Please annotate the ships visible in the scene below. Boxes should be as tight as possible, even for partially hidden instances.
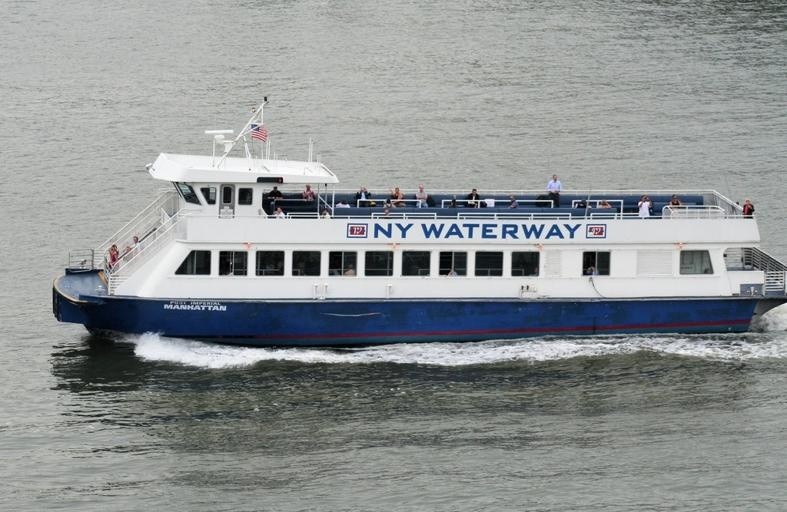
[51,92,787,347]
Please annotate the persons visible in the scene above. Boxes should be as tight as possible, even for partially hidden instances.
[577,200,587,208]
[547,175,561,207]
[586,263,599,275]
[448,200,456,208]
[743,199,755,219]
[600,201,611,208]
[448,270,457,277]
[357,187,377,206]
[319,207,330,219]
[669,193,681,219]
[415,184,428,208]
[336,200,355,208]
[638,195,653,217]
[464,189,485,208]
[344,263,355,275]
[266,186,284,211]
[509,196,518,208]
[271,207,285,220]
[302,183,316,199]
[384,188,405,208]
[107,235,141,277]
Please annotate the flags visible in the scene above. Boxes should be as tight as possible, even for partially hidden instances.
[251,123,268,143]
